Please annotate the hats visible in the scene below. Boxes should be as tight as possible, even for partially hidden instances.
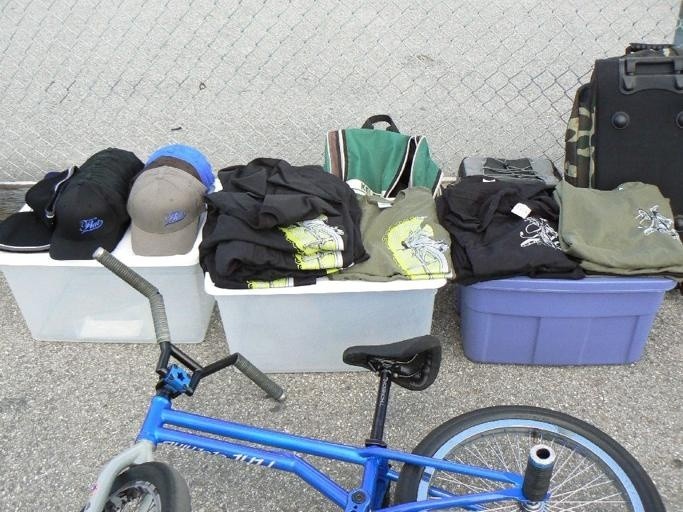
[49,181,126,260]
[126,167,200,256]
[25,164,76,241]
[129,154,202,181]
[146,143,216,188]
[71,147,144,222]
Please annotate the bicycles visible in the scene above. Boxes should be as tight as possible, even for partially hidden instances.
[82,248,671,512]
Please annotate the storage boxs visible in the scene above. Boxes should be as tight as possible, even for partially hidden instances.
[458,275,677,368]
[0,175,222,346]
[202,269,446,375]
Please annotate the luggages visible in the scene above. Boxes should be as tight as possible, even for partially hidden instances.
[565,41,683,231]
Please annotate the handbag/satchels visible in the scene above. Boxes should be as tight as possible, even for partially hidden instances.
[323,114,441,202]
